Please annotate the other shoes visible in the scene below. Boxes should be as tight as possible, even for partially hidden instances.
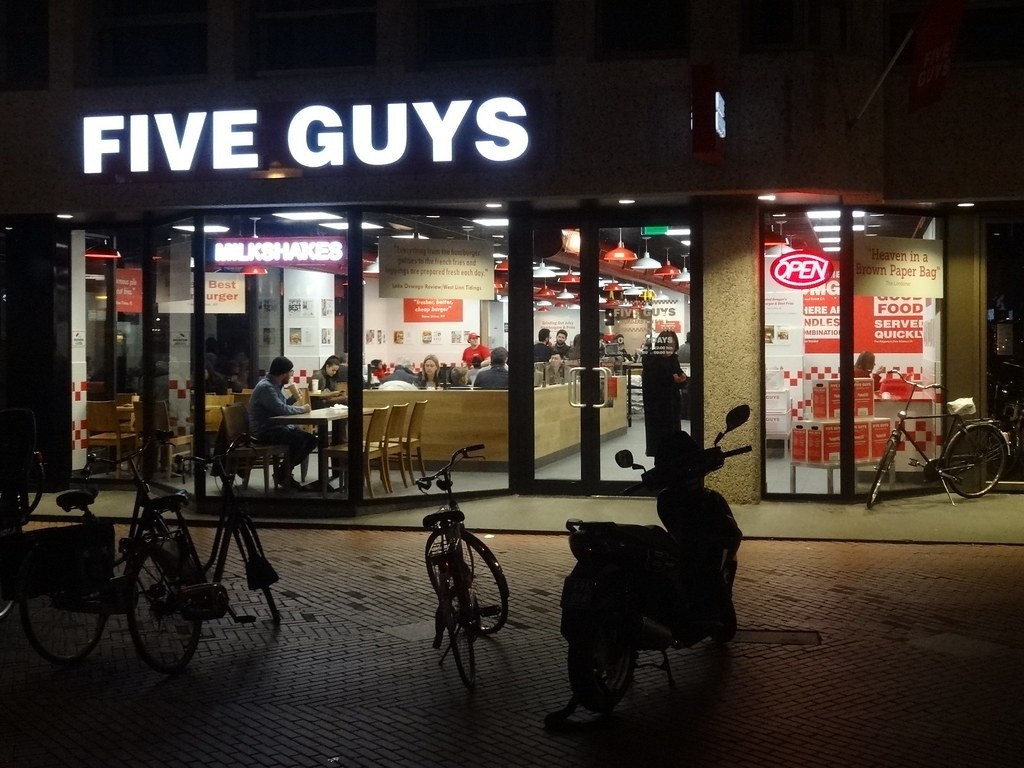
[273,468,301,492]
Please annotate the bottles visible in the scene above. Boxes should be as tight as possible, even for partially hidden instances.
[226,376,233,395]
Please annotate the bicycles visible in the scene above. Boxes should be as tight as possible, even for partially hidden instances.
[15,429,189,667]
[121,431,281,674]
[866,370,1013,507]
[414,443,510,692]
[0,441,52,623]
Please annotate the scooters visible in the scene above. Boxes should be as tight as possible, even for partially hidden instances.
[558,403,753,714]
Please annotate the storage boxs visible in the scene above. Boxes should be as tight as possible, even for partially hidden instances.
[792,378,891,463]
[765,370,792,433]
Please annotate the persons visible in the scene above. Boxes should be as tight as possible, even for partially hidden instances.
[204,353,242,395]
[599,333,627,354]
[533,329,555,362]
[379,367,422,387]
[565,333,581,360]
[249,357,316,491]
[336,354,365,386]
[854,351,883,391]
[463,332,491,369]
[643,330,686,459]
[678,332,690,362]
[88,354,126,401]
[553,330,570,358]
[467,356,489,384]
[371,360,387,378]
[450,367,469,387]
[474,347,509,387]
[545,352,564,379]
[418,355,444,386]
[310,356,345,408]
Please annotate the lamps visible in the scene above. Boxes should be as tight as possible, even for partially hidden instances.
[604,229,690,282]
[493,257,669,312]
[84,239,120,257]
[362,243,380,273]
[240,217,268,275]
[764,225,789,246]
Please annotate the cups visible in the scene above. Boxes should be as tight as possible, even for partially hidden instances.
[311,379,319,390]
[131,395,140,407]
[286,384,302,401]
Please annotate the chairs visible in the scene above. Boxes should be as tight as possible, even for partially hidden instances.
[87,382,429,498]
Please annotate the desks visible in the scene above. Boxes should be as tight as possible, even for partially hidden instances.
[790,459,896,494]
[234,391,342,399]
[874,390,933,472]
[270,407,375,497]
[116,405,224,479]
[766,435,790,460]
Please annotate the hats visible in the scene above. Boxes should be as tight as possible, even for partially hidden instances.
[269,357,294,375]
[469,333,480,339]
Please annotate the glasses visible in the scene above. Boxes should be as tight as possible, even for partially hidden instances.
[288,370,294,376]
